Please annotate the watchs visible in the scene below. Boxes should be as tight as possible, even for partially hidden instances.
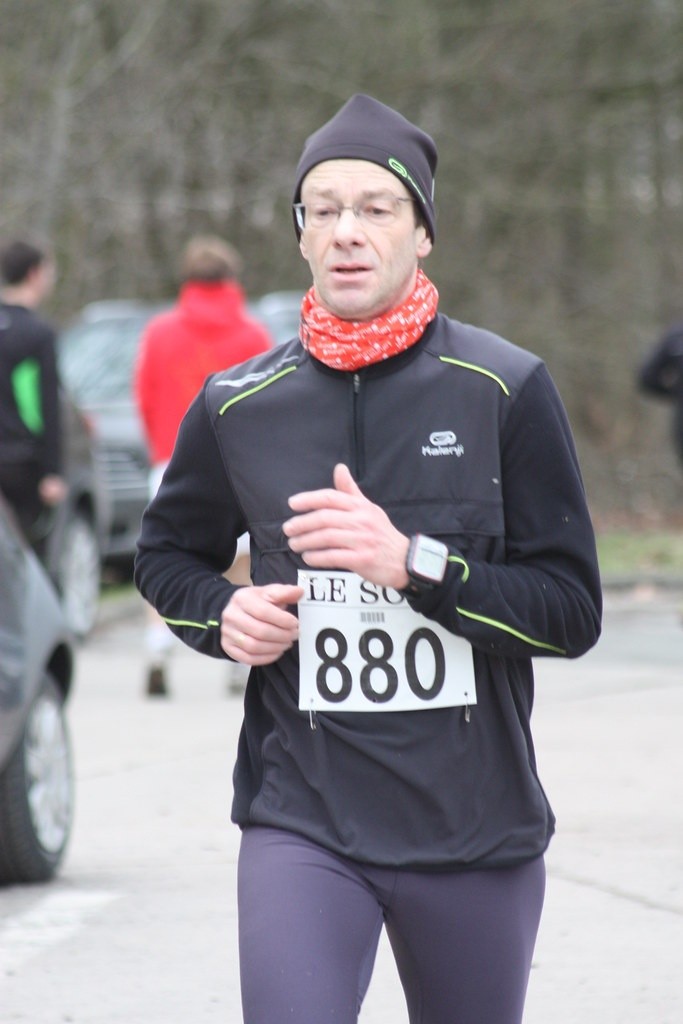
[396,532,449,604]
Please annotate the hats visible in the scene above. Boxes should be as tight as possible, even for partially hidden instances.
[291,93,438,245]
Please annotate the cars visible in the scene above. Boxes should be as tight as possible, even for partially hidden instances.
[64,299,165,578]
[2,504,88,881]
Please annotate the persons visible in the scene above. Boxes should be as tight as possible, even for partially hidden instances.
[138,228,275,694]
[637,328,683,462]
[131,90,605,1024]
[0,240,65,558]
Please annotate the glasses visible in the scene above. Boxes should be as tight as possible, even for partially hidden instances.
[293,194,417,228]
